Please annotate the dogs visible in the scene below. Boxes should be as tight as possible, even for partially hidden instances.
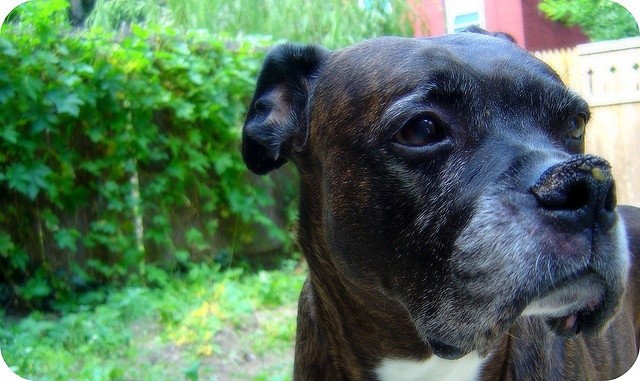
[242,26,640,380]
